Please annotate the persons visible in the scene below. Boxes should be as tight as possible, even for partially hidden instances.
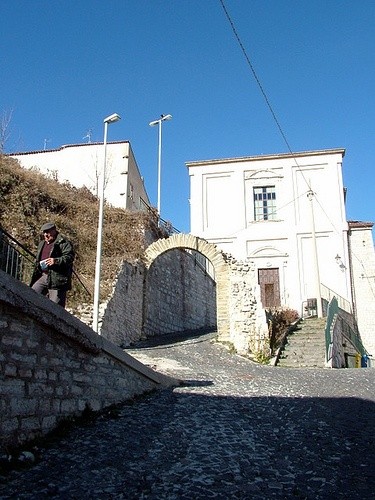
[29,222,75,309]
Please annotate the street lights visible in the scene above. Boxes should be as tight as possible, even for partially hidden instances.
[148,114,173,225]
[91,113,122,335]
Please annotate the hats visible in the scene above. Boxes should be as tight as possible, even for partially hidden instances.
[42,223,55,231]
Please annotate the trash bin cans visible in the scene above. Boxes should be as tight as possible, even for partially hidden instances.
[354,353,362,368]
[361,353,368,367]
